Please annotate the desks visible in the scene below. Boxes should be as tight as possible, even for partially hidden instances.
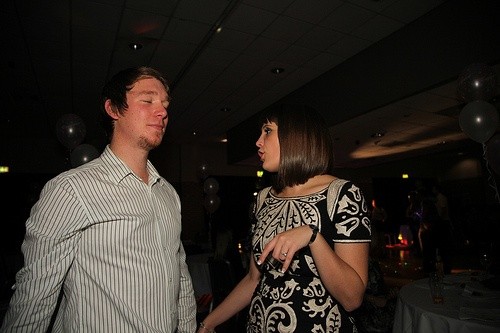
[392,274,500,333]
[186,253,213,314]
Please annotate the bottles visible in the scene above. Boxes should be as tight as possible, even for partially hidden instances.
[432,273,443,304]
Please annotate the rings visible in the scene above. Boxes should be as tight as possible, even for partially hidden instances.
[280,253,287,256]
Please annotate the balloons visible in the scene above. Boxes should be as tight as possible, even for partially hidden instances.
[195,159,209,179]
[70,143,98,168]
[457,100,500,177]
[55,112,86,146]
[202,195,221,214]
[204,178,219,196]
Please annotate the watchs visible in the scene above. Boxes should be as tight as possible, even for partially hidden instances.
[309,223,319,244]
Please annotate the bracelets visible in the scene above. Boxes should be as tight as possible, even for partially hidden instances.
[200,321,215,333]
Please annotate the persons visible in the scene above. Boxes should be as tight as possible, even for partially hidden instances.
[196,101,457,333]
[0,65,196,333]
